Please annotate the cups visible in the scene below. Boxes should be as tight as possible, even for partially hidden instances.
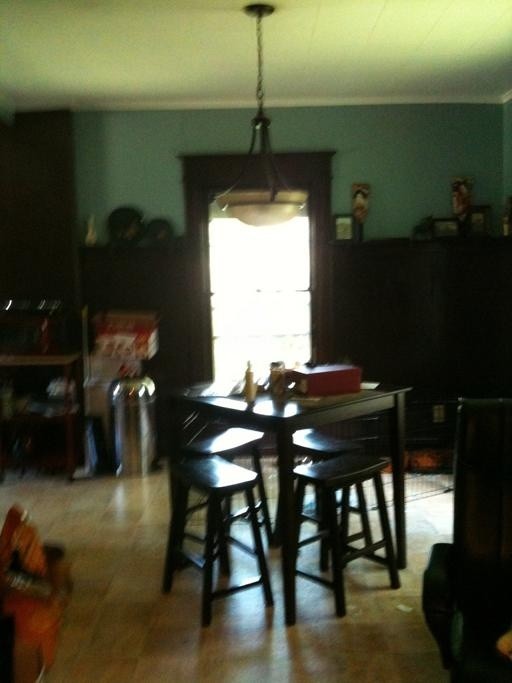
[269,361,286,401]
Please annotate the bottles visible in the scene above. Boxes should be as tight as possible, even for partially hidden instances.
[84,215,99,247]
[500,215,509,234]
[245,361,257,404]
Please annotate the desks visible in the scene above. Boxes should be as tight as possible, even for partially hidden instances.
[168,357,412,627]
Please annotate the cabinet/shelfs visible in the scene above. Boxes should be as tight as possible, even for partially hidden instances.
[0,230,164,483]
[329,241,511,444]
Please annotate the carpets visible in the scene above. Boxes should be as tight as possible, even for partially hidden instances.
[337,469,455,513]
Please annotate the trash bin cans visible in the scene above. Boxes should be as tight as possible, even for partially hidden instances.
[108,379,157,478]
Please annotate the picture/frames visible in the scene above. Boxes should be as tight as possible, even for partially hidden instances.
[431,215,462,240]
[330,213,357,242]
[464,203,494,237]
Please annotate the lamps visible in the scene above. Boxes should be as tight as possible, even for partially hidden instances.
[206,0,310,228]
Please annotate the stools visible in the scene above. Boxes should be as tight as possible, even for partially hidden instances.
[159,452,274,633]
[167,426,272,570]
[269,417,405,574]
[282,452,401,627]
